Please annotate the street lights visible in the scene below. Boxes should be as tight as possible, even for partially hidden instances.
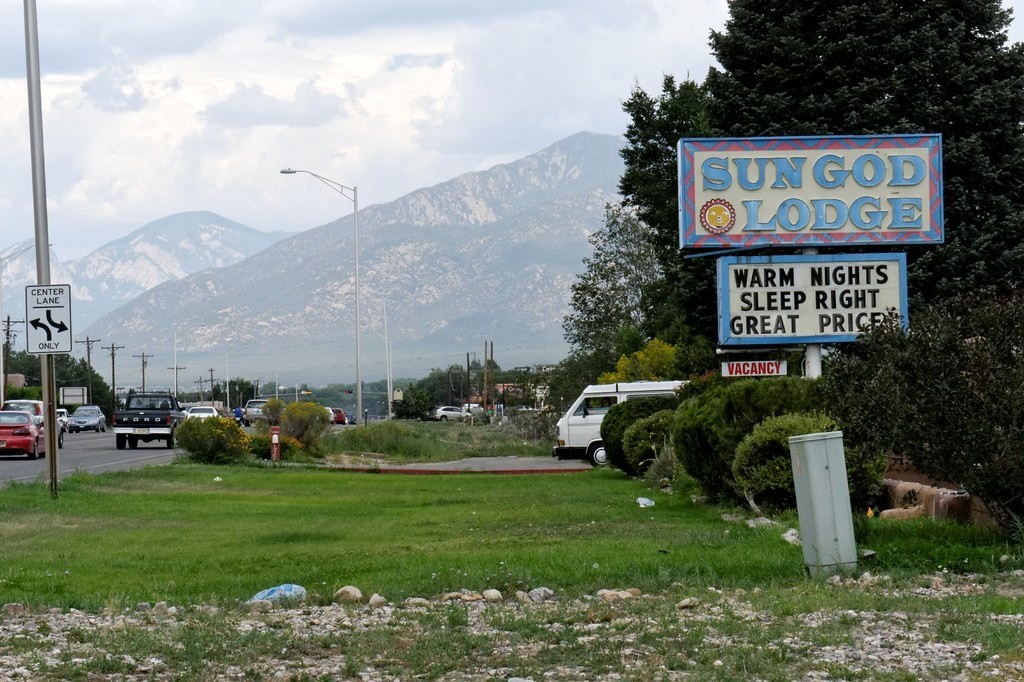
[279,167,362,425]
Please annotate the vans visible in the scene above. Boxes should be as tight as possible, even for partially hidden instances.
[551,379,691,467]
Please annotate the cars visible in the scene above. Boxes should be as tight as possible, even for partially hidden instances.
[517,405,533,410]
[429,406,471,422]
[324,406,337,425]
[0,399,64,448]
[332,407,347,425]
[56,405,107,433]
[0,410,45,460]
[188,407,221,423]
[244,399,278,426]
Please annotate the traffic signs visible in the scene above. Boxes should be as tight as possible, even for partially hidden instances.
[25,283,74,355]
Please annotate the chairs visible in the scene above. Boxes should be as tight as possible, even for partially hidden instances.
[15,417,25,423]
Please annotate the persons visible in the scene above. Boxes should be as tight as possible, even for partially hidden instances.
[235,407,245,425]
[601,397,612,408]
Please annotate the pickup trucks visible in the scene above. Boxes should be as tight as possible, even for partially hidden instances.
[111,391,187,450]
